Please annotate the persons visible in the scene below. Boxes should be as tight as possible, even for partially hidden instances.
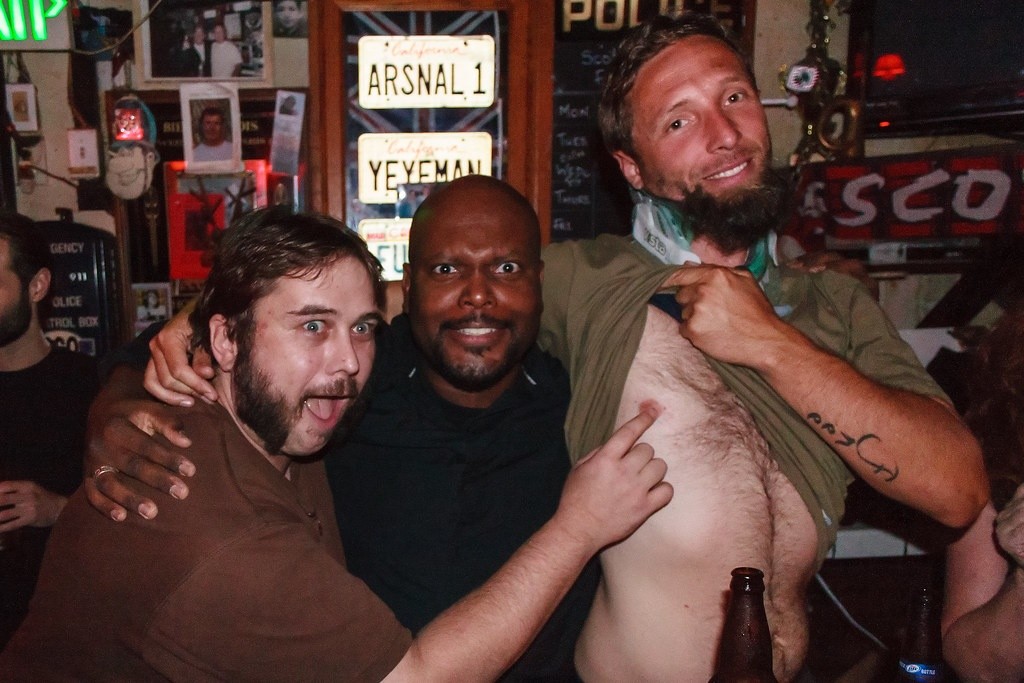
[144,15,989,683]
[83,17,134,91]
[194,107,232,162]
[85,176,879,681]
[114,112,139,134]
[274,0,306,36]
[46,336,81,352]
[182,23,243,78]
[399,185,430,218]
[0,207,675,683]
[941,306,1024,683]
[0,213,104,654]
[139,292,164,321]
[281,95,297,116]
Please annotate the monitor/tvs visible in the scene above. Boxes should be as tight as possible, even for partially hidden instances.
[845,0,1024,139]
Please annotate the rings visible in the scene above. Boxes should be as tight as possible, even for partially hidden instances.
[93,464,120,483]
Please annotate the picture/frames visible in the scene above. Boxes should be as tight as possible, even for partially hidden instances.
[132,0,275,90]
[6,84,38,132]
[179,83,242,173]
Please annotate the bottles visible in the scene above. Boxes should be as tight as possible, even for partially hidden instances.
[893,577,944,683]
[708,566,782,683]
